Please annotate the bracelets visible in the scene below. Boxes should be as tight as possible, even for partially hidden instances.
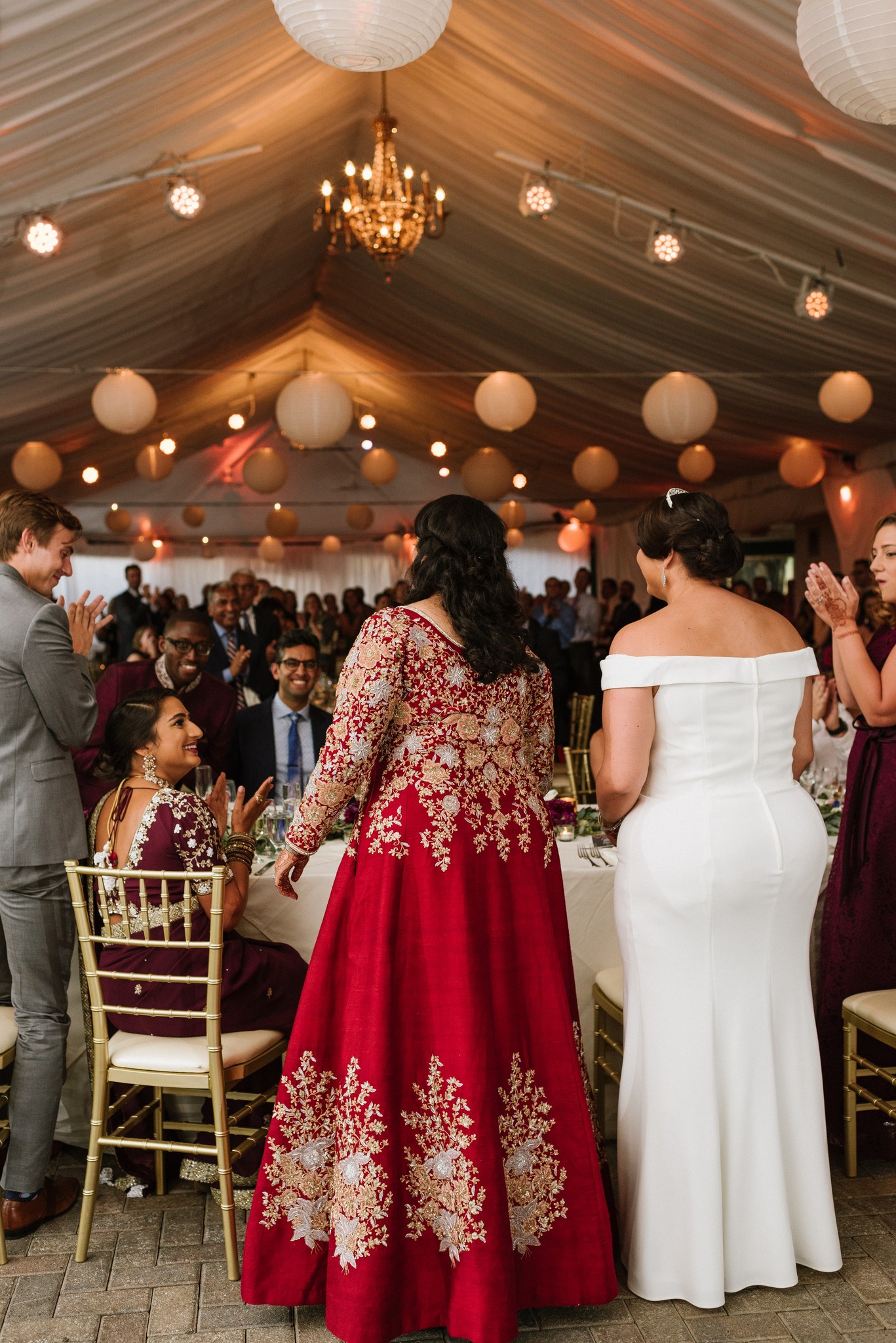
[224,832,258,875]
[597,812,625,832]
[284,842,299,855]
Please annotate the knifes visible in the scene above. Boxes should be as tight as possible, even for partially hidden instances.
[253,861,275,876]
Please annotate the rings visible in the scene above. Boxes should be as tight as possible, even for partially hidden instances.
[273,875,276,879]
[816,594,824,602]
[254,794,264,804]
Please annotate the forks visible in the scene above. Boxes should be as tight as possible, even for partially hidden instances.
[589,843,615,868]
[577,843,601,867]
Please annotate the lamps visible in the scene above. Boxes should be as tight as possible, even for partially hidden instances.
[311,70,453,288]
[514,168,558,221]
[643,218,684,268]
[164,172,206,220]
[12,209,63,261]
[793,273,837,323]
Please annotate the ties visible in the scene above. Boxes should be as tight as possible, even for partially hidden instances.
[226,629,247,710]
[242,613,252,633]
[574,596,580,612]
[277,713,304,839]
[600,601,611,624]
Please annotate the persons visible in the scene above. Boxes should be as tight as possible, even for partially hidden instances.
[84,688,310,1211]
[0,490,118,1241]
[804,511,896,1163]
[595,490,848,1309]
[72,560,888,819]
[239,494,621,1343]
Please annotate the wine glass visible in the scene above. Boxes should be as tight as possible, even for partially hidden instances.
[799,755,819,794]
[820,767,840,809]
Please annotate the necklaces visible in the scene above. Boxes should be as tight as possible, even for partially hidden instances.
[132,775,175,790]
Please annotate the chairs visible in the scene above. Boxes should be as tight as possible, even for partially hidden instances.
[838,982,896,1189]
[61,857,288,1285]
[538,689,597,804]
[587,964,634,1164]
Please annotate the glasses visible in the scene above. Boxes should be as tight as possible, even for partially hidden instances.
[163,635,212,657]
[278,658,318,672]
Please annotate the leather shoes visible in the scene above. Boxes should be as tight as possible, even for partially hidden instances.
[2,1176,78,1241]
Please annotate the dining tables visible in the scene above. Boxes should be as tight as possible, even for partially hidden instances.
[186,792,656,1094]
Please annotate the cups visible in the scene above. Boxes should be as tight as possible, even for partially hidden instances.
[556,825,575,842]
[196,764,356,857]
